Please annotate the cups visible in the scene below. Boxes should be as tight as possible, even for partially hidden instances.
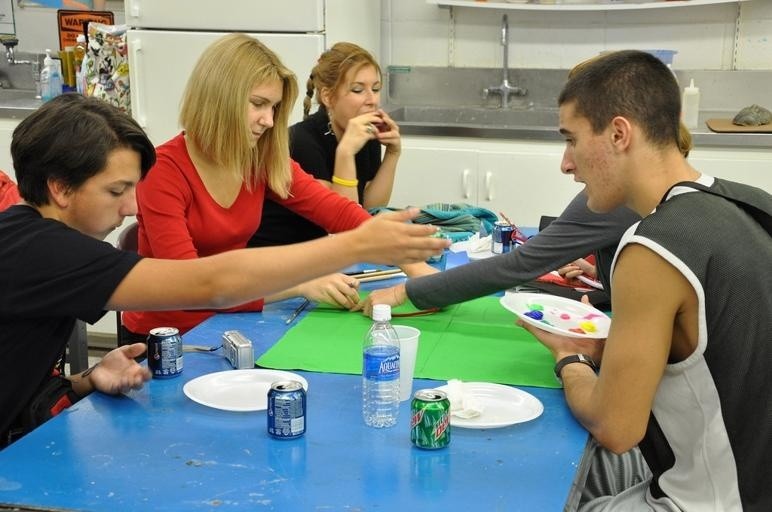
[372,325,420,402]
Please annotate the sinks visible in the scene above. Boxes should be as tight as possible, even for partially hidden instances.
[386,106,561,129]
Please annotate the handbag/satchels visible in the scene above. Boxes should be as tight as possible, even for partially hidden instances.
[24,376,76,434]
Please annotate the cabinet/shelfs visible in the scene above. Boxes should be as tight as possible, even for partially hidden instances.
[678,149,771,195]
[376,141,594,234]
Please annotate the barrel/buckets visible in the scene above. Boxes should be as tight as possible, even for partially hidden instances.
[599,49,680,89]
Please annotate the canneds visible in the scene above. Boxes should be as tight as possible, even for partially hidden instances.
[491,221,517,254]
[410,389,451,449]
[266,380,307,440]
[146,327,184,379]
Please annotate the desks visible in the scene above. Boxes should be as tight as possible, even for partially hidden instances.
[1,221,612,510]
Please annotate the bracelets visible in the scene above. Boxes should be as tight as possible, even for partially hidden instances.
[331,174,363,190]
[548,352,597,379]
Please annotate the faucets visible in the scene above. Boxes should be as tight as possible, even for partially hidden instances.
[2,38,42,98]
[481,13,528,109]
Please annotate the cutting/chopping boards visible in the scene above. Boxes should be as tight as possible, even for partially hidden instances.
[706,116,772,133]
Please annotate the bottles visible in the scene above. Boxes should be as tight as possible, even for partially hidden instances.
[73,34,88,73]
[679,78,700,133]
[364,302,402,424]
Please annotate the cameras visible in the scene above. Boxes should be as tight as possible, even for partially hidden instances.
[222,330,254,370]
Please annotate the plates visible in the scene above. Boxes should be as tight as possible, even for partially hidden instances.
[184,368,311,413]
[499,290,612,340]
[430,379,544,433]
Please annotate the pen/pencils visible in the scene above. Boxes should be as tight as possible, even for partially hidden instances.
[286,299,310,325]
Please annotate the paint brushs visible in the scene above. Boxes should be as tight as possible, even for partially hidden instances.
[499,210,527,239]
[344,269,407,282]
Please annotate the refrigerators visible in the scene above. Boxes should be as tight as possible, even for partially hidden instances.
[126,0,383,152]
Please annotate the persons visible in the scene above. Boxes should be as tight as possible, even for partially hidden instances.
[348,120,772,321]
[1,90,453,445]
[246,40,401,244]
[118,32,445,364]
[516,49,771,511]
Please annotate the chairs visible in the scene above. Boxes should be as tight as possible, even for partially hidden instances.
[113,223,144,366]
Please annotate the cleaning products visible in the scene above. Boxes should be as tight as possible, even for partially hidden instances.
[57,34,87,89]
[682,78,700,131]
